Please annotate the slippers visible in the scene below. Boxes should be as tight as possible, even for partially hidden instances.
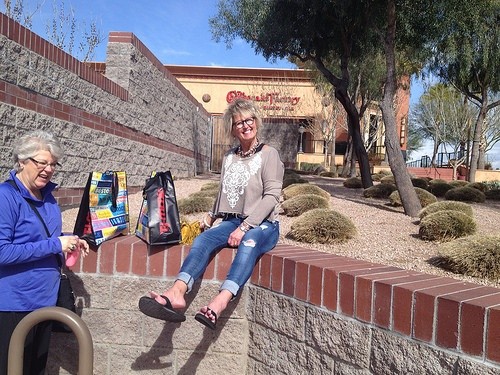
[139,295,186,322]
[195,306,217,330]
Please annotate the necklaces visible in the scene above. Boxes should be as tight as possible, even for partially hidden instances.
[238,141,259,158]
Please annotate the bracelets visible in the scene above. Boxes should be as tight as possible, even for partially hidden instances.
[203,216,212,228]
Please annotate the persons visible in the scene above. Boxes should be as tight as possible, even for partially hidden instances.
[0,129,91,375]
[138,98,283,329]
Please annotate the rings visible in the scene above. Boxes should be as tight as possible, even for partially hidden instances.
[67,249,71,253]
[71,246,75,250]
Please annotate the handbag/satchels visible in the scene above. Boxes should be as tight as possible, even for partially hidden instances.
[74,170,130,246]
[135,171,182,245]
[50,270,78,334]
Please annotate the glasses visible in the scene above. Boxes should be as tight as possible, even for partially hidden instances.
[29,158,62,171]
[232,117,255,128]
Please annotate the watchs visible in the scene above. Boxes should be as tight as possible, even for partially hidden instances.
[237,224,248,233]
